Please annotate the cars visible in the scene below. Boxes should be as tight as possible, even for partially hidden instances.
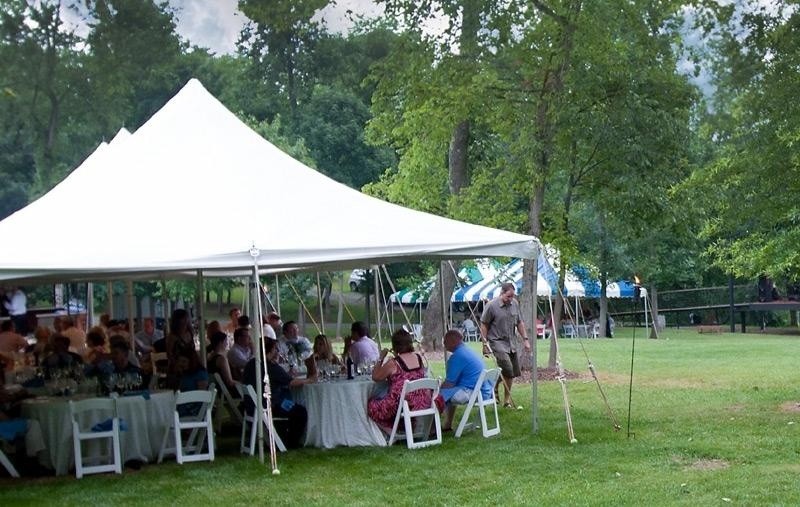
[450,301,485,312]
[348,267,374,293]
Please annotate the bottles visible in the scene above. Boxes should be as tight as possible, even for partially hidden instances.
[346,351,354,380]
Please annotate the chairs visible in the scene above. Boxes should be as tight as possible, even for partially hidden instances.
[388,375,442,449]
[402,324,599,342]
[67,393,122,480]
[456,365,502,438]
[151,352,288,465]
[0,449,21,477]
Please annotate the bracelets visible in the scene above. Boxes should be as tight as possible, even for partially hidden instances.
[378,359,383,362]
[523,337,528,342]
[482,342,488,345]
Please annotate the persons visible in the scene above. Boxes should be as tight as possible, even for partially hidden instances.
[368,328,445,445]
[343,321,378,374]
[243,336,318,448]
[688,310,695,326]
[535,302,615,338]
[447,303,482,341]
[165,308,346,417]
[479,283,531,409]
[429,329,493,436]
[41,318,163,395]
[2,320,56,477]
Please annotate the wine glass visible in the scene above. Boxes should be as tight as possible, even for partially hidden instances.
[318,360,375,382]
[27,361,141,398]
[276,350,308,374]
